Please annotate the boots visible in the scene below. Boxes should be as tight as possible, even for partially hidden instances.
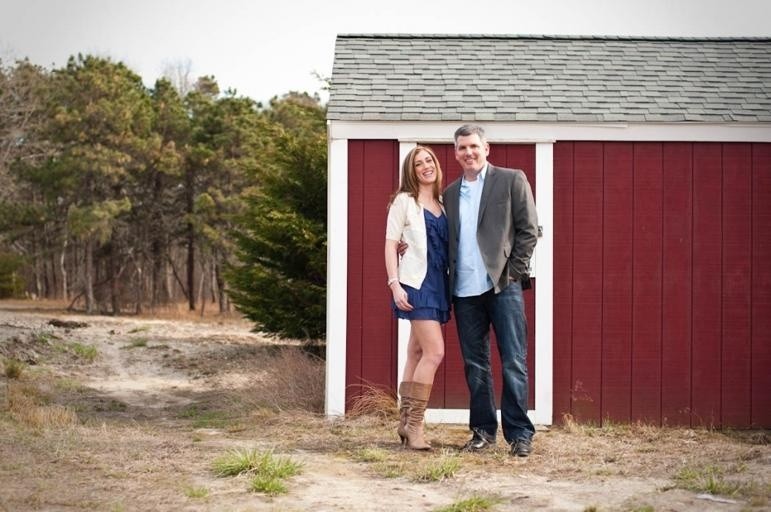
[398,381,432,452]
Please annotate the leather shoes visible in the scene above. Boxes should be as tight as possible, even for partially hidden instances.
[464,438,495,451]
[511,441,530,456]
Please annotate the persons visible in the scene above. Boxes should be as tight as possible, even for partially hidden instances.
[395,122,542,459]
[381,143,454,453]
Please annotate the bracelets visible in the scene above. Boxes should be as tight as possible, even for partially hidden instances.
[387,277,399,287]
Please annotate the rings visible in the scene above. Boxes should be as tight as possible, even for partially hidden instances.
[397,304,401,309]
